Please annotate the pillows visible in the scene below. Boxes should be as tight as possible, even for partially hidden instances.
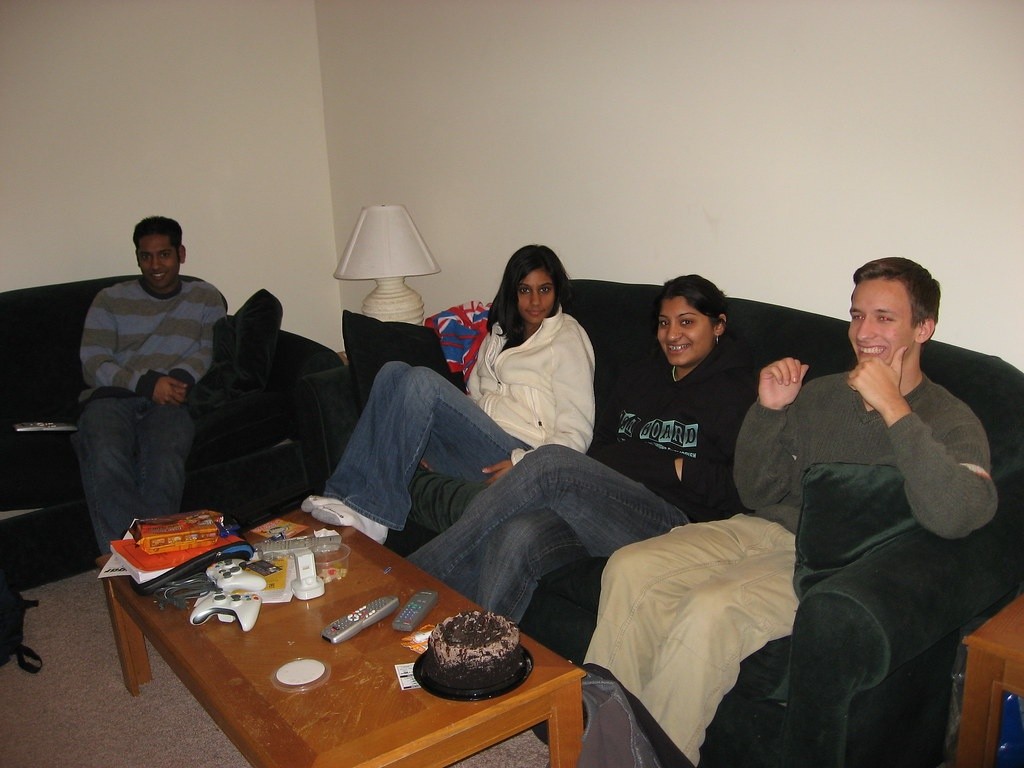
[792,461,916,600]
[186,289,283,418]
[342,310,452,415]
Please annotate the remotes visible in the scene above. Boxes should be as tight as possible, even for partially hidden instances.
[250,535,342,561]
[13,422,79,433]
[392,589,440,632]
[320,595,399,644]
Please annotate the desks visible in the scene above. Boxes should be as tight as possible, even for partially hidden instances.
[955,594,1024,768]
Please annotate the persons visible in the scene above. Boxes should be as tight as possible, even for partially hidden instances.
[70,217,226,555]
[584,257,998,768]
[405,275,760,625]
[300,245,595,544]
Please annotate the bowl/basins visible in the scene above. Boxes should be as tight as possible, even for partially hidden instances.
[308,542,352,583]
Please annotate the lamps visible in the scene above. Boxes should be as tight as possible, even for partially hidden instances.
[333,205,442,326]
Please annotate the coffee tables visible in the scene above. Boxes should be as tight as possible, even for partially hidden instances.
[95,510,587,768]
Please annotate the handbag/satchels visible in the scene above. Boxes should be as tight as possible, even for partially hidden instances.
[0,570,26,668]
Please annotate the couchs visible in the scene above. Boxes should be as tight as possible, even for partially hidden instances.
[287,279,1024,768]
[0,274,342,592]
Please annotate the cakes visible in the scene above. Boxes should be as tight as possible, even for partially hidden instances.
[426,610,521,688]
